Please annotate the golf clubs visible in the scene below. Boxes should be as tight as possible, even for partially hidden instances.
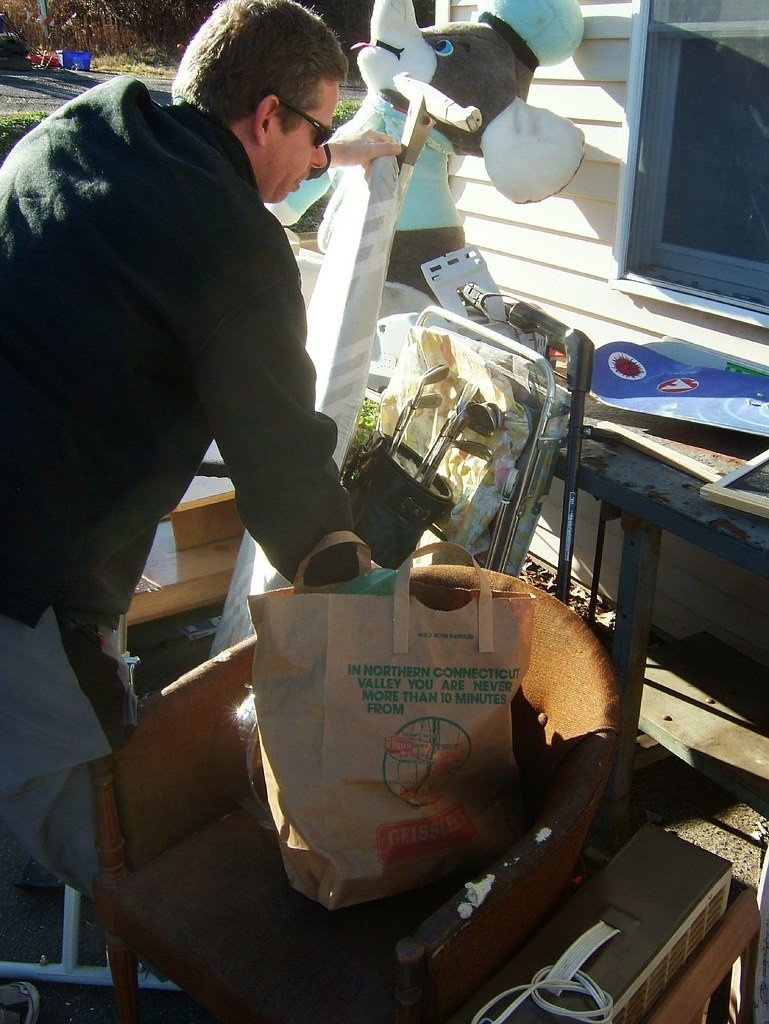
[386,361,506,489]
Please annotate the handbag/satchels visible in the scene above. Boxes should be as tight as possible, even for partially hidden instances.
[246,530,535,909]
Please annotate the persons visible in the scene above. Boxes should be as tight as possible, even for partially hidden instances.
[0,0,403,902]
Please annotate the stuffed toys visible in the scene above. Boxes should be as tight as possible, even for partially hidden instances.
[262,0,586,307]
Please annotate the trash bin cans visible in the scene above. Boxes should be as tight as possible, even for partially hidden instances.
[56,50,93,71]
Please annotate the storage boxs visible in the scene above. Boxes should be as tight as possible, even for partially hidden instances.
[55,50,94,71]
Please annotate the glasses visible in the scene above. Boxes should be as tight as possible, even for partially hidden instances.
[261,89,336,149]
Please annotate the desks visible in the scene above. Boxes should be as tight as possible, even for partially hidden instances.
[126,521,243,623]
[556,447,769,846]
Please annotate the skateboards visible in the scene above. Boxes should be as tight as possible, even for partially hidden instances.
[590,340,769,437]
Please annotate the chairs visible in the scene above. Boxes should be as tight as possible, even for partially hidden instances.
[87,564,624,1024]
